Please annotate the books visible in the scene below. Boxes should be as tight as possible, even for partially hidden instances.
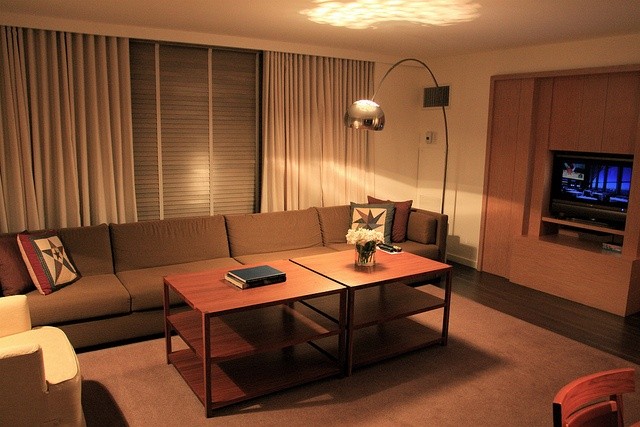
[224,264,286,288]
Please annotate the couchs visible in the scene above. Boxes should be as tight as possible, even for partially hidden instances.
[2,195,449,355]
[0,293,89,424]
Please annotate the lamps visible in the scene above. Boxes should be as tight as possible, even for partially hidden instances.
[342,57,450,217]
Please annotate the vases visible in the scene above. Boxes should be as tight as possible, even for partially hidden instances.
[355,243,375,266]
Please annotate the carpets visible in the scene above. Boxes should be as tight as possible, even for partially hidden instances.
[75,282,640,427]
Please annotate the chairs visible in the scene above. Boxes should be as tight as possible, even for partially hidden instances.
[551,369,640,425]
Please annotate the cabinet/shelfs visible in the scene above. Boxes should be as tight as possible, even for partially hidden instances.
[508,216,639,319]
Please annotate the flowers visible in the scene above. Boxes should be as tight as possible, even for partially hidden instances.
[345,229,384,246]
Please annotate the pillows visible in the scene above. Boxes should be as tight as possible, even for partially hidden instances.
[17,229,81,295]
[350,200,396,246]
[366,195,413,244]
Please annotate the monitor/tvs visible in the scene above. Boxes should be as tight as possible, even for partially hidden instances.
[549,151,633,227]
